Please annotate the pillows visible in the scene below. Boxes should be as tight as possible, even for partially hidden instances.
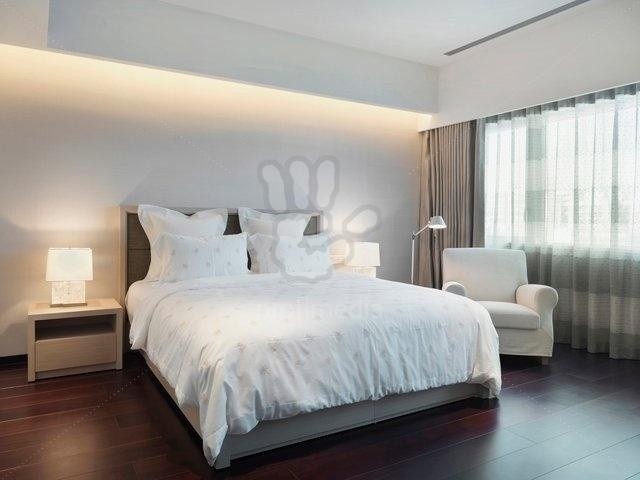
[136,204,334,287]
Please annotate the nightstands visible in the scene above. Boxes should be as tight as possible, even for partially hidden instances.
[27,298,124,382]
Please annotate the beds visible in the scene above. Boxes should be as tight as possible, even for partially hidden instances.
[118,204,502,472]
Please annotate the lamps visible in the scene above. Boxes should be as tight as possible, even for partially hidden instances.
[342,240,381,279]
[45,248,94,307]
[409,216,447,285]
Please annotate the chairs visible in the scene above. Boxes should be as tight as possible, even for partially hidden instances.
[441,247,559,366]
